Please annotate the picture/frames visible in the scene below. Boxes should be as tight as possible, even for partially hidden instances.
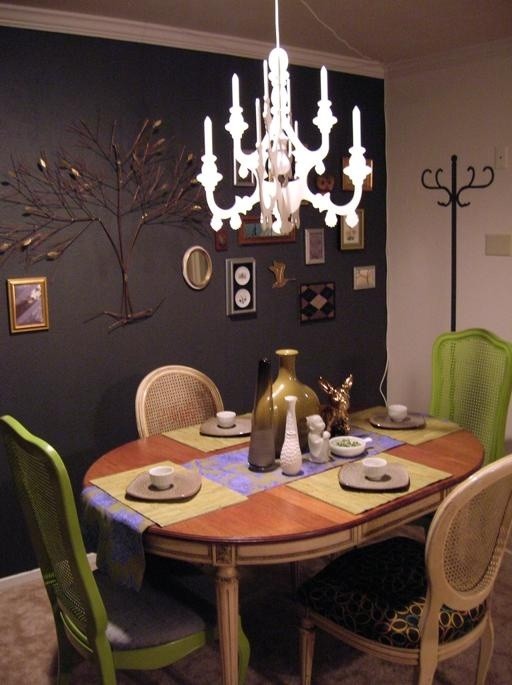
[237,216,297,246]
[300,282,335,324]
[342,156,372,193]
[231,149,257,188]
[225,256,258,321]
[353,265,376,290]
[7,276,50,335]
[304,228,325,265]
[340,209,364,251]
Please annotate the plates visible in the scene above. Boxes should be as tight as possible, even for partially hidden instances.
[234,266,251,309]
[339,462,410,489]
[126,471,202,501]
[369,413,425,429]
[200,416,252,436]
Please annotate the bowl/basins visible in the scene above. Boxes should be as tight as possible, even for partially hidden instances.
[329,436,372,458]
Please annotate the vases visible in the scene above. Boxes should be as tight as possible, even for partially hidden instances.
[279,396,302,475]
[252,350,323,458]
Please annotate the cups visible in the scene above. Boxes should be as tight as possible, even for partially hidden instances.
[388,405,408,422]
[216,411,236,427]
[362,458,387,481]
[149,466,174,490]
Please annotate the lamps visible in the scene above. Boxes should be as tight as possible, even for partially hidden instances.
[194,0,373,235]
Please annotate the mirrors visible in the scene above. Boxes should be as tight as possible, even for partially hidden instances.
[182,245,212,290]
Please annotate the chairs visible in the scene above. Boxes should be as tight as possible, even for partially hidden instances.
[292,455,512,684]
[2,416,250,685]
[135,364,225,439]
[429,328,512,468]
